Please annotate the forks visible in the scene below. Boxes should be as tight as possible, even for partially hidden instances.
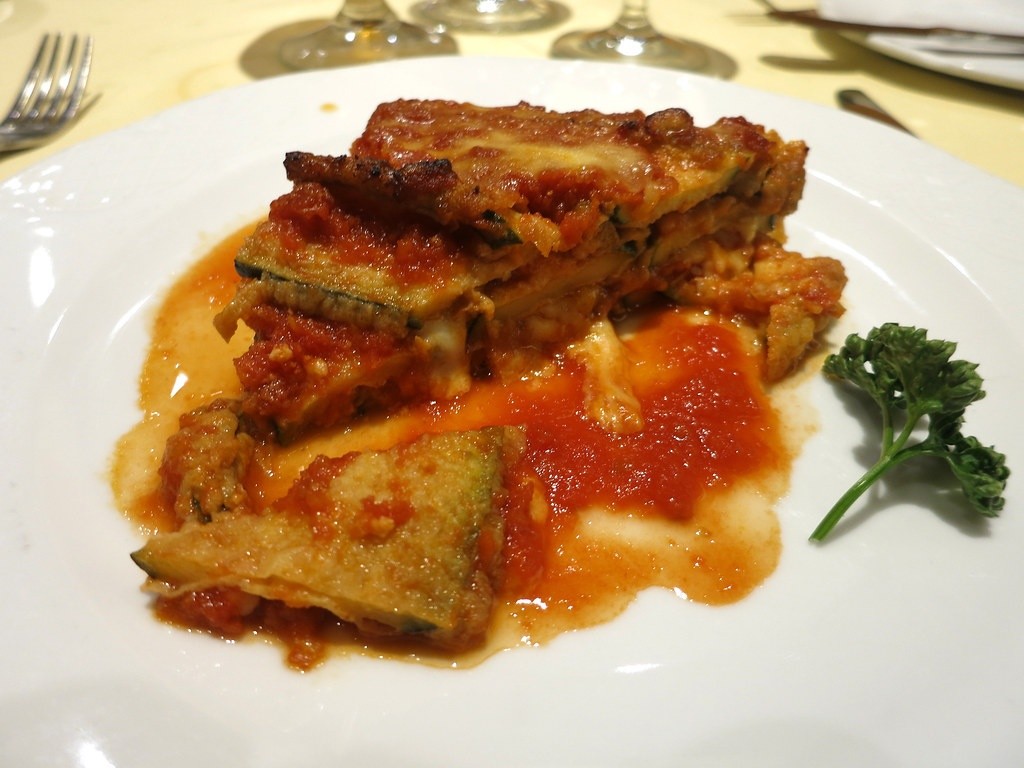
[1,31,94,154]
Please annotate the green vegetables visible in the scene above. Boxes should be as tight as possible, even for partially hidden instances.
[806,321,1010,542]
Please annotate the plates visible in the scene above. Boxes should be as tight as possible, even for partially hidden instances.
[1,54,1021,767]
[831,24,1024,93]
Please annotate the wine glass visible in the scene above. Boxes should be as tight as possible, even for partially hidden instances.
[239,0,739,79]
